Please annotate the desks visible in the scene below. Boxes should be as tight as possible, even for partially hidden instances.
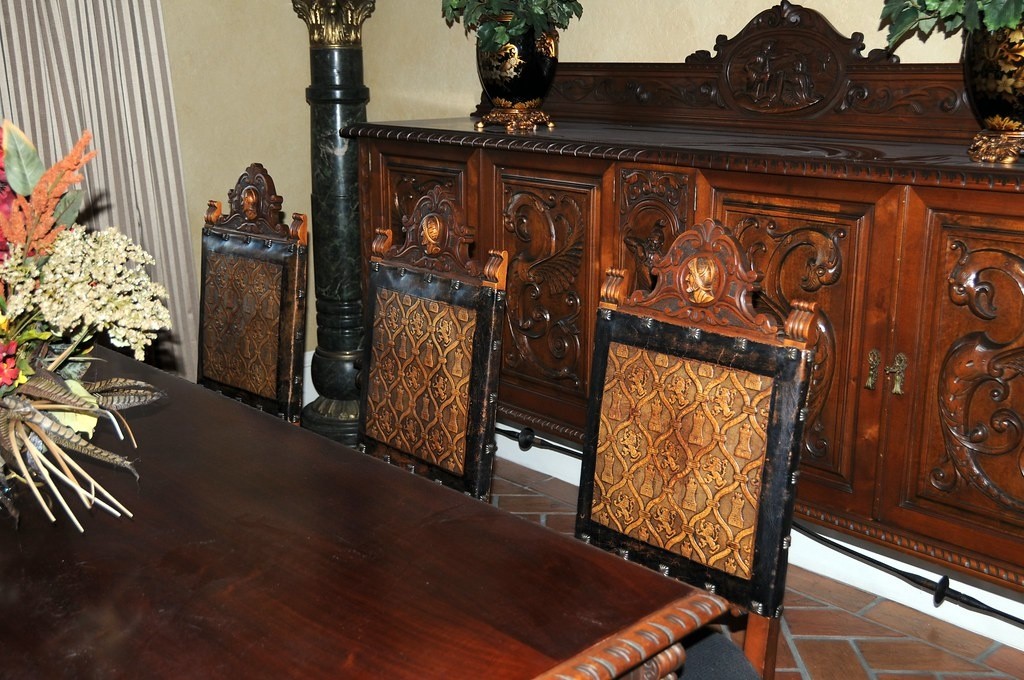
[0,343,728,680]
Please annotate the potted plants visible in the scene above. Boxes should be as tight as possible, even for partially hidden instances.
[878,0,1024,163]
[442,0,583,133]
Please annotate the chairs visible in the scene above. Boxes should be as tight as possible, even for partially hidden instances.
[196,162,311,426]
[356,183,508,499]
[574,218,821,680]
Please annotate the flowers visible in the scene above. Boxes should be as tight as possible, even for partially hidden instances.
[0,120,175,532]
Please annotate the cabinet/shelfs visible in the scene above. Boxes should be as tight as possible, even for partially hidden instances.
[340,0,1024,594]
